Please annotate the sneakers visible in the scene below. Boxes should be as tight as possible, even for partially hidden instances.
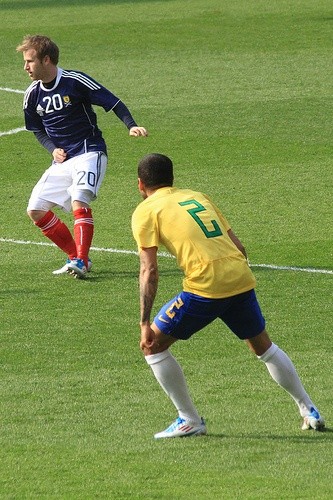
[52,259,93,275]
[302,411,324,431]
[153,416,207,438]
[68,258,87,279]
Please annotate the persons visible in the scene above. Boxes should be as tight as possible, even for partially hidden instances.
[17,33,149,278]
[131,153,328,439]
